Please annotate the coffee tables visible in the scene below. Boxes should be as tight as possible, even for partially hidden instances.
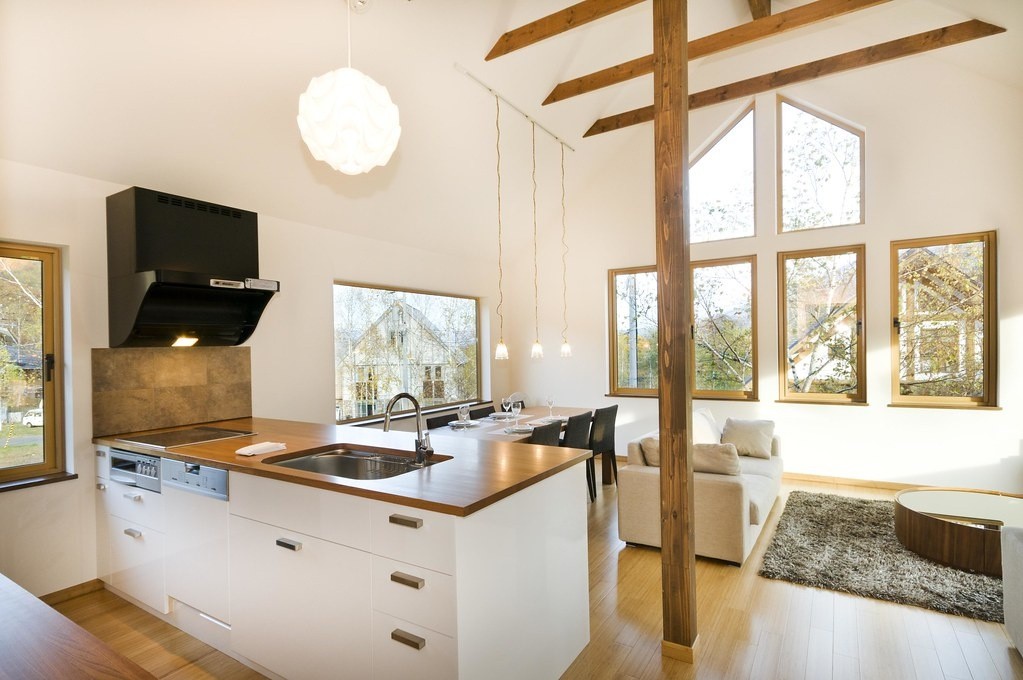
[894,487,1023,577]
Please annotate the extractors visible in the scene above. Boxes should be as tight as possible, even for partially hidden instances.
[103,187,280,349]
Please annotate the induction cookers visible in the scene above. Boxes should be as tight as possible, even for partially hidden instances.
[106,424,258,454]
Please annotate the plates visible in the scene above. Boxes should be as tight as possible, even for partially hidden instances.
[489,411,519,418]
[504,426,536,433]
[448,419,479,427]
[539,416,570,422]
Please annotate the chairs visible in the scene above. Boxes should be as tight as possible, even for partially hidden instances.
[589,404,617,497]
[468,405,496,420]
[526,420,562,446]
[426,413,459,430]
[501,400,527,413]
[559,410,595,503]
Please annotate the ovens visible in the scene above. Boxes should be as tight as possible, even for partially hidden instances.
[94,442,170,626]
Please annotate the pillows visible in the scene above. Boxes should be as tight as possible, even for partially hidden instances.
[693,442,742,476]
[721,416,774,461]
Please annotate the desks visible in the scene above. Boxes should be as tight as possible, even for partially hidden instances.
[0,573,159,680]
[421,405,615,485]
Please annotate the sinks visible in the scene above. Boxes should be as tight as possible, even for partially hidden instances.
[270,450,427,480]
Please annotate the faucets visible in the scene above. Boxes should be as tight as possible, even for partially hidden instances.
[384,392,434,463]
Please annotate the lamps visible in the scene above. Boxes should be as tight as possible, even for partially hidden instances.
[495,89,509,360]
[559,142,573,358]
[296,0,401,176]
[530,121,543,357]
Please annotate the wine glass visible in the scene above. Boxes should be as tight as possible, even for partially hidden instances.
[459,404,471,432]
[546,396,555,419]
[509,402,522,429]
[502,396,512,422]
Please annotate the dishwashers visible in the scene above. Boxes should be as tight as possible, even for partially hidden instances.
[165,448,229,656]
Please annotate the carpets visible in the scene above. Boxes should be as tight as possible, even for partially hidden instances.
[759,490,1007,628]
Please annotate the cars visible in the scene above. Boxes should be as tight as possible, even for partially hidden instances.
[22,410,44,428]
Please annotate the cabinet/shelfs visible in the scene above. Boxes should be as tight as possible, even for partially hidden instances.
[94,441,591,680]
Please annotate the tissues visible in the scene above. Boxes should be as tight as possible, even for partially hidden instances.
[501,392,525,412]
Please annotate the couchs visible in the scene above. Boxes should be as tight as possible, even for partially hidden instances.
[617,408,782,568]
